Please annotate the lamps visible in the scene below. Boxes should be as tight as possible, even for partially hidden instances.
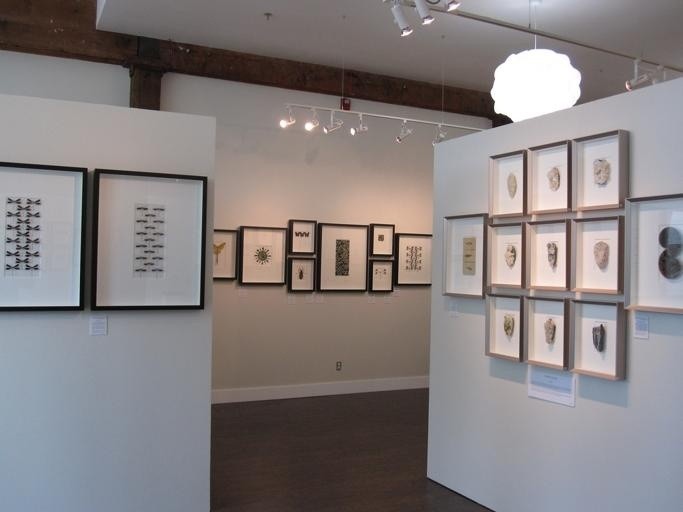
[490,0,585,128]
[278,101,488,145]
[618,48,670,92]
[385,0,463,38]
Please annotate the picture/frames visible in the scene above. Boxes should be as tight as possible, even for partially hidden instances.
[211,216,432,297]
[0,160,88,312]
[84,164,209,316]
